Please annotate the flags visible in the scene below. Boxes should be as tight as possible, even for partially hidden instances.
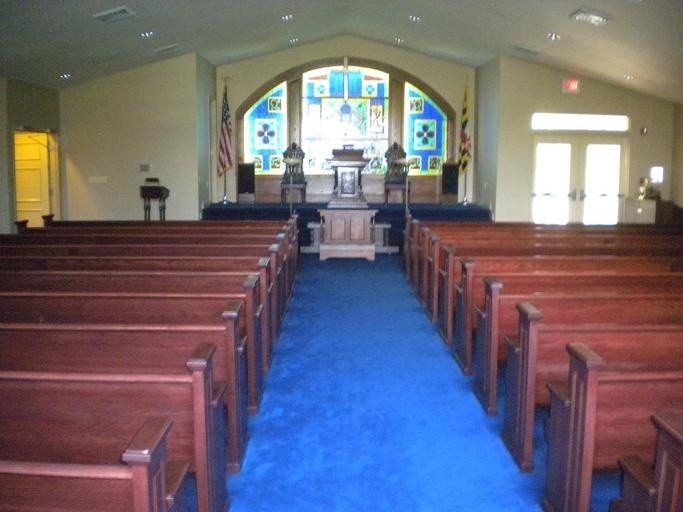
[457,86,473,176]
[216,83,234,177]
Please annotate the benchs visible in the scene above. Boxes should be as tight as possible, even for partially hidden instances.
[0,212,300,511]
[402,214,683,512]
